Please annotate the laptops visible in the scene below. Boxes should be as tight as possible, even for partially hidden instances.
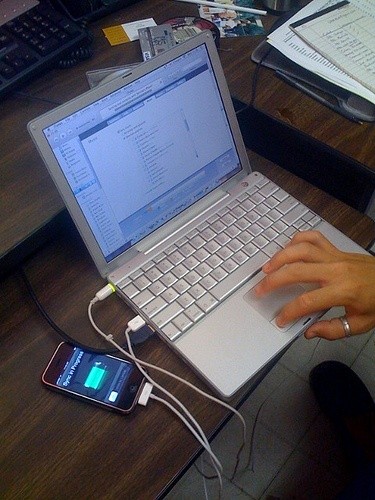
[27,29,372,402]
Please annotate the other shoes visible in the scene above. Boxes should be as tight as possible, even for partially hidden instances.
[311,361,375,450]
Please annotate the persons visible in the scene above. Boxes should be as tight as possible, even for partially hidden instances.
[251,230,375,500]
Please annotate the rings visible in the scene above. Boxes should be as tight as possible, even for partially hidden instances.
[340,316,352,337]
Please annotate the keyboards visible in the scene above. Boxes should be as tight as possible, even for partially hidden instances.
[0,3,89,99]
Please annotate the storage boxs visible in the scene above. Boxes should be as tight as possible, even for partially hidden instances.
[138,24,175,62]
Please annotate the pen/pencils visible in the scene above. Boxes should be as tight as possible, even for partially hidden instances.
[178,0,267,16]
[275,70,362,124]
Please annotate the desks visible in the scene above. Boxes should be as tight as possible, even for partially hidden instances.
[1,0,375,500]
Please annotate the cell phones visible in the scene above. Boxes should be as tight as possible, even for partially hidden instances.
[41,340,149,414]
